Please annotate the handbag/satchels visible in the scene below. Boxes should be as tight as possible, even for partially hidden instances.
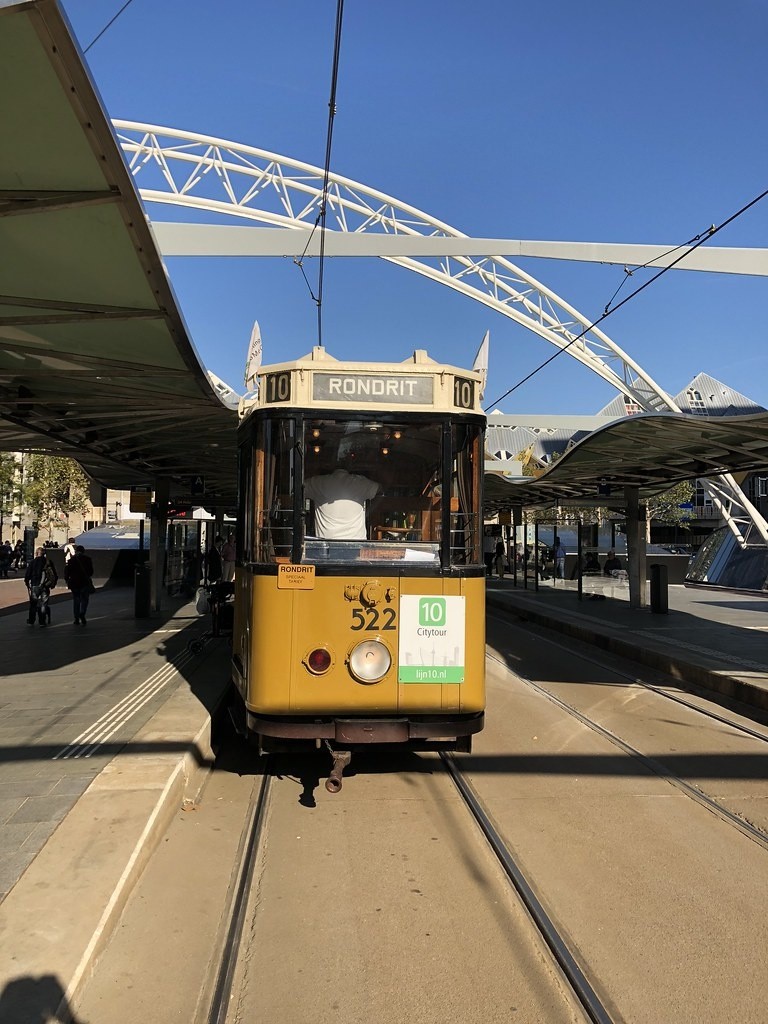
[501,554,510,565]
[83,583,97,595]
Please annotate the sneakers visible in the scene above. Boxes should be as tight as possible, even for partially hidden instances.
[39,623,46,628]
[26,618,34,626]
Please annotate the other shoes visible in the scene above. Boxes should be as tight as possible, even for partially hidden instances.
[497,576,505,580]
[557,576,564,579]
[74,616,87,625]
[553,575,558,578]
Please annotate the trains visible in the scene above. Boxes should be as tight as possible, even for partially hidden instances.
[232,320,489,793]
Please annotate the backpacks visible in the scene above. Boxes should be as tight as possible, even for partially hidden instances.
[39,560,58,589]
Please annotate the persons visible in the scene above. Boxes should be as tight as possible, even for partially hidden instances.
[43,540,59,555]
[572,552,601,580]
[63,538,78,564]
[221,535,236,581]
[483,530,496,576]
[204,535,225,615]
[14,539,22,569]
[65,546,94,625]
[24,548,58,627]
[0,541,18,578]
[303,450,394,541]
[552,537,565,578]
[496,542,505,580]
[604,551,622,579]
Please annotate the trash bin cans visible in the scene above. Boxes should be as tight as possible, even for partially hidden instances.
[134,569,151,620]
[650,564,668,614]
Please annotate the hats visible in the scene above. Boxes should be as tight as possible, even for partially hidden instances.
[497,536,503,543]
